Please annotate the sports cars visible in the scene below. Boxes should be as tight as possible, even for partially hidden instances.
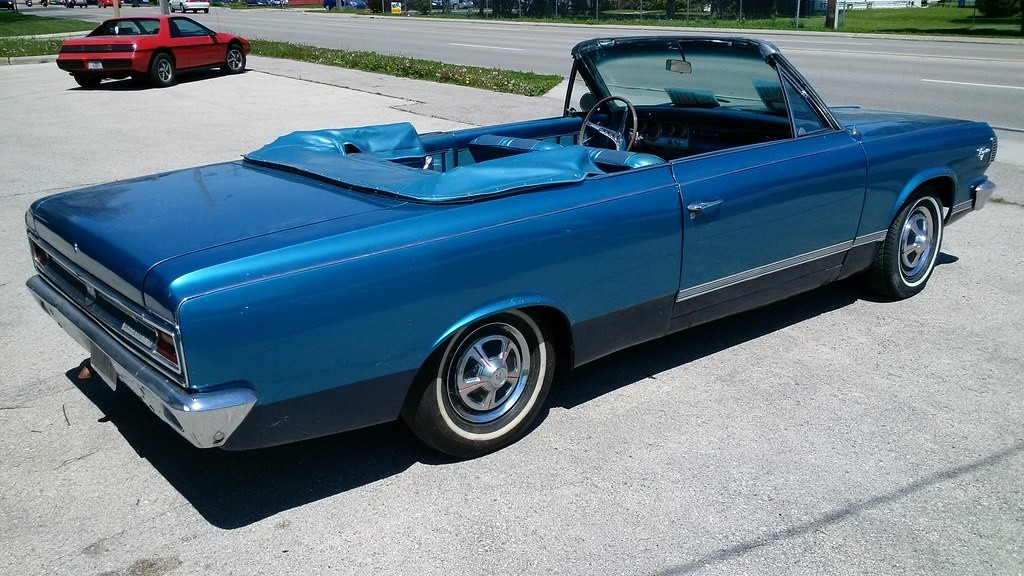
[21,32,1000,464]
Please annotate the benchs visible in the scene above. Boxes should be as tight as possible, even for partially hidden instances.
[467,134,669,170]
[240,121,604,205]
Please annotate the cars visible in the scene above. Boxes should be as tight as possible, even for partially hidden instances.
[168,0,210,14]
[322,0,368,12]
[56,14,252,89]
[432,0,475,11]
[66,0,88,8]
[247,0,288,7]
[98,0,122,9]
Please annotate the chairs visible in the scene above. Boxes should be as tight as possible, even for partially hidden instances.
[153,27,160,35]
[117,27,136,35]
